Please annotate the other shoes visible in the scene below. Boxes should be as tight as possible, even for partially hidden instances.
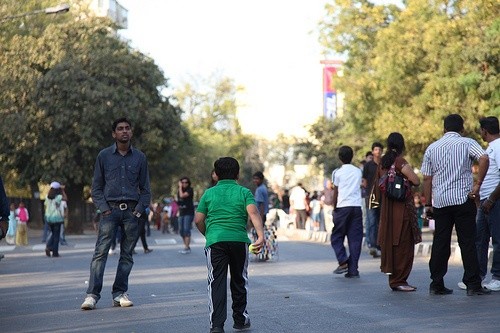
[430,286,453,294]
[108,249,115,254]
[144,249,152,253]
[345,271,359,277]
[46,249,50,257]
[52,255,60,257]
[467,287,491,296]
[178,247,191,254]
[210,327,224,333]
[392,285,417,291]
[233,319,250,329]
[333,263,348,274]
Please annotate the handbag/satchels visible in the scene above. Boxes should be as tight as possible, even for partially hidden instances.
[386,174,407,200]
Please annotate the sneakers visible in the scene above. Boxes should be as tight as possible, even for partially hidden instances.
[112,292,133,306]
[484,279,500,291]
[458,278,486,289]
[81,296,96,309]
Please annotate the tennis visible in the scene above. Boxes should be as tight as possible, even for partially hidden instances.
[249,244,259,254]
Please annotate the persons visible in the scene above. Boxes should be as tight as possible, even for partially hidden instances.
[207,168,219,189]
[178,176,194,254]
[16,201,29,245]
[1,177,10,259]
[145,195,178,237]
[360,142,384,258]
[365,152,374,163]
[81,118,152,309]
[421,114,492,296]
[40,181,68,257]
[6,202,17,244]
[359,159,367,172]
[375,132,420,292]
[194,157,264,333]
[330,146,364,278]
[458,116,500,291]
[266,182,334,232]
[248,171,269,262]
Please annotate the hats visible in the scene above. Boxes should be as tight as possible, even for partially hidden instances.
[51,182,61,188]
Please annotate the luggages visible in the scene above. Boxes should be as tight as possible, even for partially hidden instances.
[251,226,277,261]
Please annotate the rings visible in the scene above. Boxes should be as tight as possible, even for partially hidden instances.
[473,194,475,198]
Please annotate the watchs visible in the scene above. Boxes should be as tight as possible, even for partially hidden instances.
[133,210,141,218]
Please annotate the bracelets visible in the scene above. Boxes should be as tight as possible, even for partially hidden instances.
[487,197,495,203]
[424,203,432,206]
